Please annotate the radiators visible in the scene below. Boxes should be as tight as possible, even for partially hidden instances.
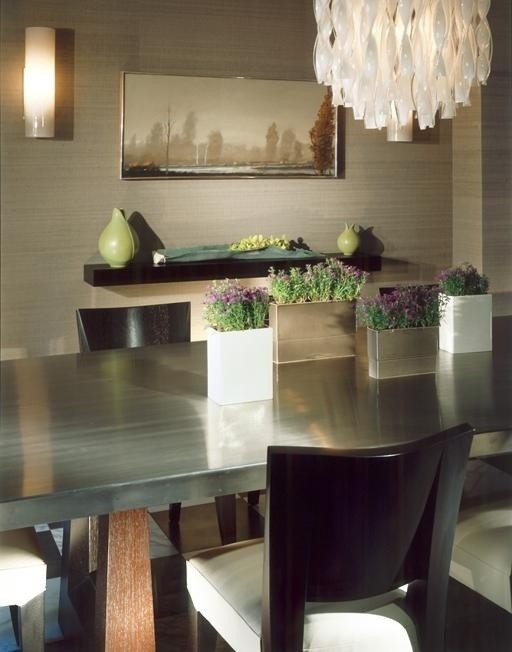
[437,292,494,355]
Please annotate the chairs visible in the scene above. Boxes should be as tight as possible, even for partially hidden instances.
[75,300,238,556]
[449,497,510,651]
[0,525,51,652]
[181,424,478,651]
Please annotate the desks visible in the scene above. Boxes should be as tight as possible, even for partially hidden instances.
[0,315,512,652]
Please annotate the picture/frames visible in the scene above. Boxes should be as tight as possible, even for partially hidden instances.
[119,69,345,183]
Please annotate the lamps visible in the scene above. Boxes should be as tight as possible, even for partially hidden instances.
[19,25,58,139]
[309,1,493,144]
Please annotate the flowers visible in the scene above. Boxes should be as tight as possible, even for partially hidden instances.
[433,262,490,297]
[267,258,371,305]
[355,281,448,331]
[200,278,271,331]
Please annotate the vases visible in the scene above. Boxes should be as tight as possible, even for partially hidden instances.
[206,324,275,408]
[337,221,364,258]
[269,299,358,367]
[366,325,442,380]
[96,206,142,268]
